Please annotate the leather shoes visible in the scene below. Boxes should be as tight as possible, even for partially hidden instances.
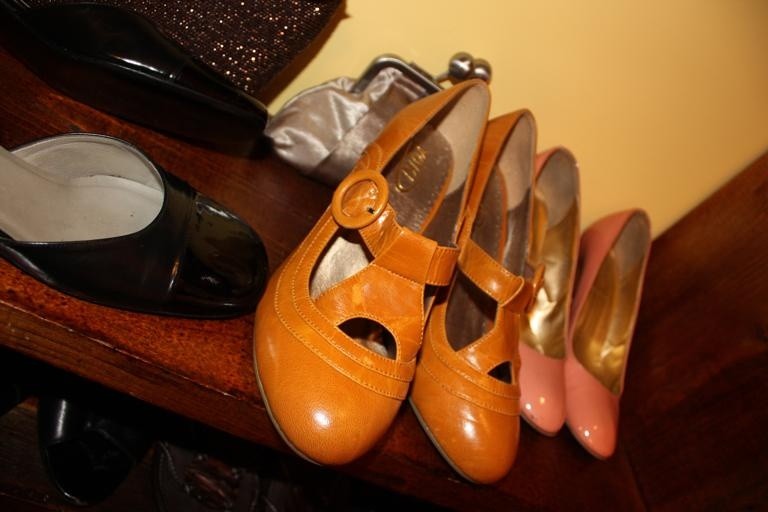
[0,133,270,319]
[515,146,583,437]
[409,108,540,487]
[38,392,150,508]
[249,79,493,468]
[563,208,652,461]
[2,0,268,146]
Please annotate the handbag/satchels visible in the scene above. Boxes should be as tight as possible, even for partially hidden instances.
[263,47,492,190]
[145,440,270,512]
[97,0,345,100]
